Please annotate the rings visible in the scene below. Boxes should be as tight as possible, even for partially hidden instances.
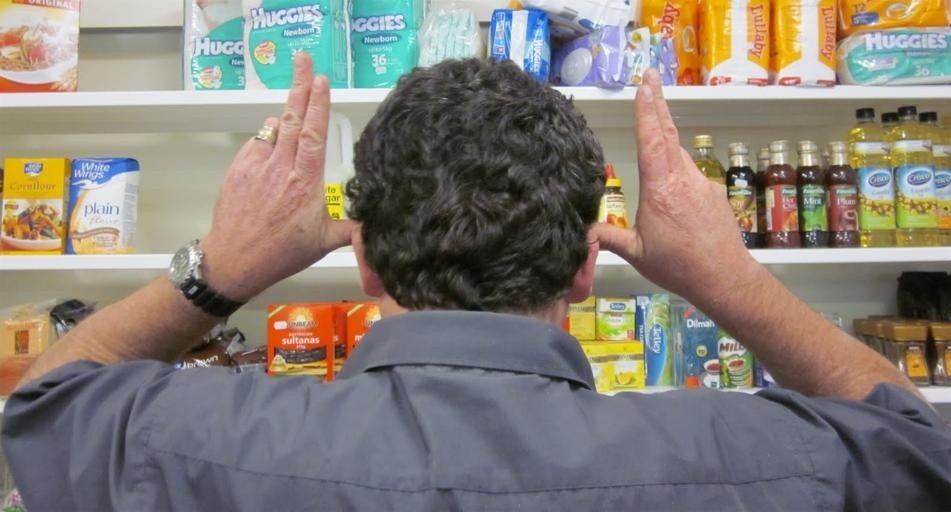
[255,125,280,146]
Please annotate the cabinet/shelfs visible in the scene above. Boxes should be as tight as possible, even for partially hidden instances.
[0,0,950,428]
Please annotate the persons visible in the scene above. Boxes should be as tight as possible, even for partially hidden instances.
[0,48,950,509]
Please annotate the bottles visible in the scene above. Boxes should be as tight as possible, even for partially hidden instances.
[66,155,140,253]
[825,142,858,248]
[848,108,896,247]
[726,142,758,249]
[1,157,71,255]
[861,319,906,359]
[597,163,628,228]
[756,146,769,249]
[691,134,726,183]
[765,139,800,249]
[795,140,827,248]
[919,110,951,246]
[883,323,932,387]
[929,322,951,386]
[889,104,935,246]
[852,317,865,346]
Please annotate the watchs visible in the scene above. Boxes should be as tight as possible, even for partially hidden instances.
[163,239,248,319]
